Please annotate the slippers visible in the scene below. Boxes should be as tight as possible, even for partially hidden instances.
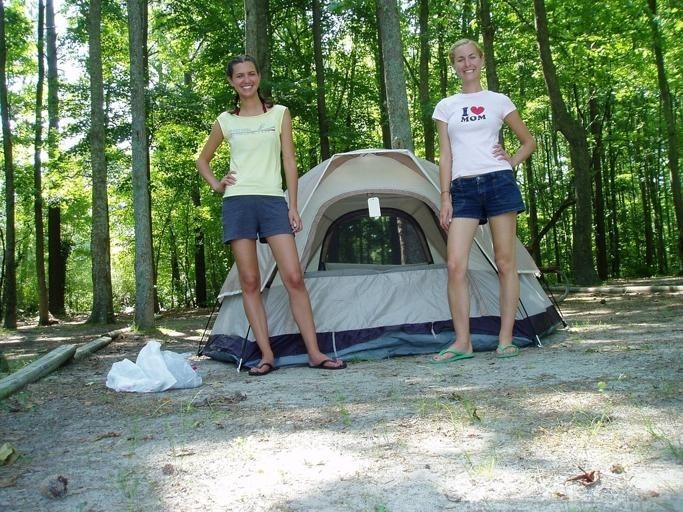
[496,343,520,359]
[249,363,280,377]
[429,348,474,365]
[308,359,348,370]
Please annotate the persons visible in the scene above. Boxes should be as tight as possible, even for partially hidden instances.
[428,39,536,364]
[195,55,346,375]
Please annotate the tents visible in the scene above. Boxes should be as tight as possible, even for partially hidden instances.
[197,149,565,371]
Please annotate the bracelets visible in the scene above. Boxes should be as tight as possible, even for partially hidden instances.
[441,191,451,196]
